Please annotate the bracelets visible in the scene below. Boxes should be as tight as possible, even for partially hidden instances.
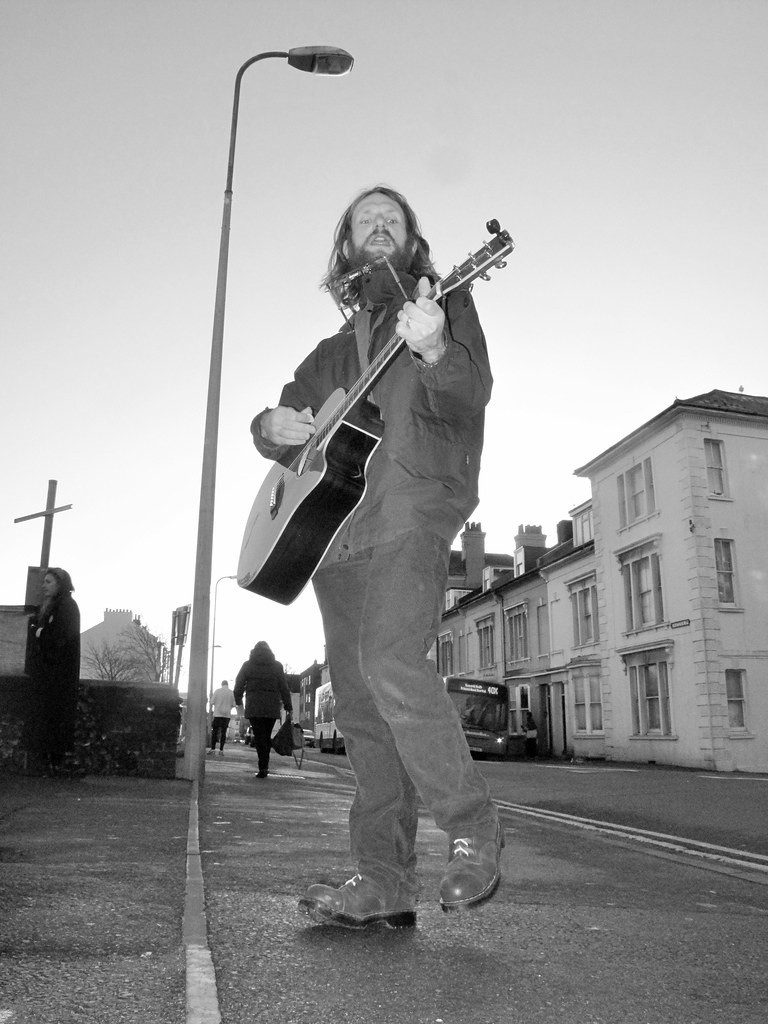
[30,624,35,628]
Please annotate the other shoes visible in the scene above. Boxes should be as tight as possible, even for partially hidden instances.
[218,751,224,756]
[207,750,214,755]
[255,769,269,778]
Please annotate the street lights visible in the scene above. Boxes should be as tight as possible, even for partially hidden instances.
[178,45,356,782]
[207,574,241,749]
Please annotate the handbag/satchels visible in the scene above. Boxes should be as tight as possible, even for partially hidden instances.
[272,714,293,756]
[292,723,304,750]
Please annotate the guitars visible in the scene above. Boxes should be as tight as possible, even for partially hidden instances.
[235,217,517,608]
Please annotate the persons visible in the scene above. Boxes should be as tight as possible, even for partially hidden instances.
[521,712,537,760]
[234,640,292,779]
[20,567,78,778]
[207,680,236,756]
[250,187,505,931]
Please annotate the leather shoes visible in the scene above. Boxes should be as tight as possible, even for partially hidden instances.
[299,872,416,931]
[438,815,505,913]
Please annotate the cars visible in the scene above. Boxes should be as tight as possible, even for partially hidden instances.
[302,727,314,749]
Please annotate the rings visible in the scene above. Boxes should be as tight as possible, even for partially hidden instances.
[406,317,412,328]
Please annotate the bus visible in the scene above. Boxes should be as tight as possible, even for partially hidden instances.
[313,681,344,754]
[441,675,507,758]
